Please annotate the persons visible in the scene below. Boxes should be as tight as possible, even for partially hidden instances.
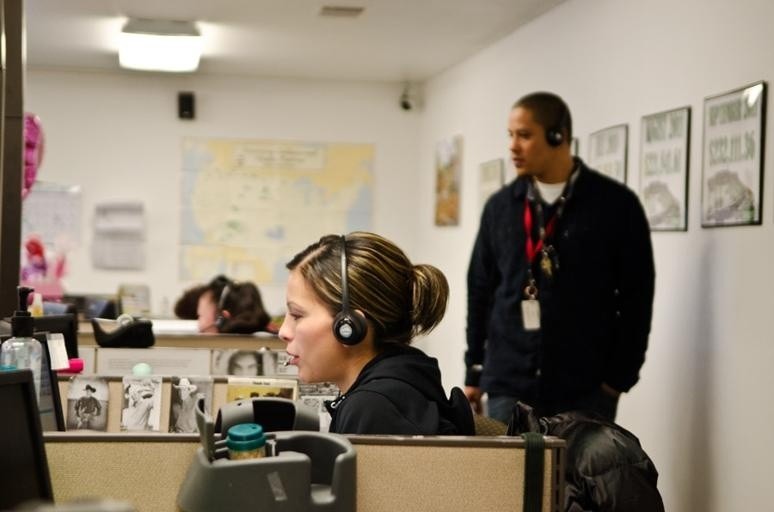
[73,385,103,429]
[463,93,654,434]
[197,276,270,334]
[121,379,157,432]
[169,378,204,433]
[278,232,449,436]
[228,350,264,376]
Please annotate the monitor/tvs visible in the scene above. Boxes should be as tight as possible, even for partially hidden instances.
[0,370,55,512]
[85,299,116,320]
[0,332,66,432]
[42,301,78,332]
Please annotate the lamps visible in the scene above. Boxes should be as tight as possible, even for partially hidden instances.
[117,18,203,73]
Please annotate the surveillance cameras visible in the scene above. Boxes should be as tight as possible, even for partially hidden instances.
[400,94,411,110]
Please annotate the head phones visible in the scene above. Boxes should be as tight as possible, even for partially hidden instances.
[546,104,566,146]
[331,235,368,344]
[213,282,233,331]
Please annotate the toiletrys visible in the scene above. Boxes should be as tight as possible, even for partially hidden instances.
[0,285,67,432]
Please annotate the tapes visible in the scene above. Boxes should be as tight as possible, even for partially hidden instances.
[94,314,136,328]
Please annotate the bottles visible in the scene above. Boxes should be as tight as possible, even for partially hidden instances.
[1,316,43,412]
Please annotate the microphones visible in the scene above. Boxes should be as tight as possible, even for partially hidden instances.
[283,356,293,367]
[199,320,215,333]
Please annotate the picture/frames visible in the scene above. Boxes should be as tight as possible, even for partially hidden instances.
[638,106,691,232]
[587,123,628,187]
[700,79,767,227]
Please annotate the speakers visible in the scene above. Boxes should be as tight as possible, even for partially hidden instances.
[178,92,194,120]
